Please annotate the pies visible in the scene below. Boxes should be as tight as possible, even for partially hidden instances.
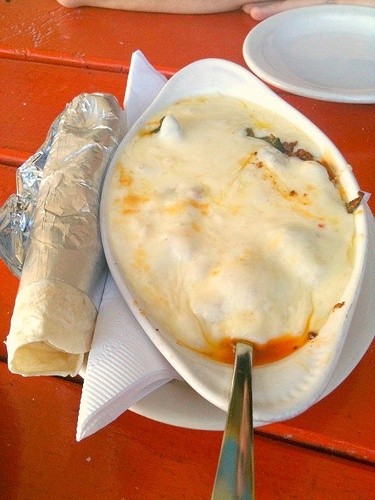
[3,280,98,378]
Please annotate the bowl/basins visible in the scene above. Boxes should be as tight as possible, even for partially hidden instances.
[99,58,369,422]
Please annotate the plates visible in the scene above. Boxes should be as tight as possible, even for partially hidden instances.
[242,4,375,104]
